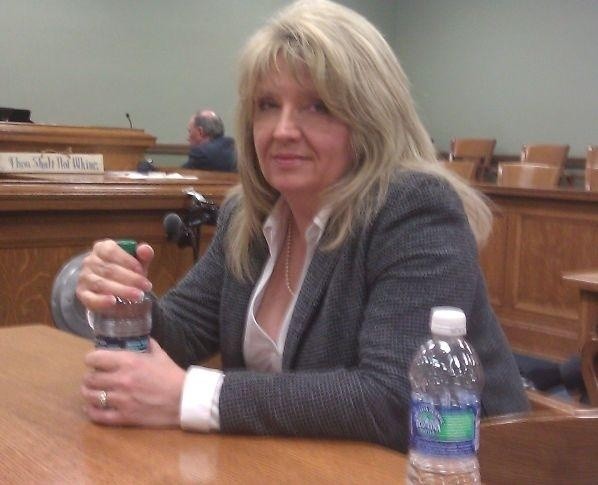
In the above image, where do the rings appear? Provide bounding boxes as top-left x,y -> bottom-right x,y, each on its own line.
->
98,389 -> 107,408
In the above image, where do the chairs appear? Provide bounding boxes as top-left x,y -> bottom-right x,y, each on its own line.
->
452,136 -> 497,180
442,155 -> 485,180
584,145 -> 598,190
0,121 -> 156,171
495,162 -> 563,189
520,144 -> 570,166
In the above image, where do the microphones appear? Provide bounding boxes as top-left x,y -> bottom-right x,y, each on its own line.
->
126,113 -> 132,128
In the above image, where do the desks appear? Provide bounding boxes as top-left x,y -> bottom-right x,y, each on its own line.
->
562,269 -> 598,409
0,325 -> 409,484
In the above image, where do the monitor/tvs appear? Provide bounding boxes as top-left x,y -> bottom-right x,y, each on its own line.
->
0,107 -> 31,123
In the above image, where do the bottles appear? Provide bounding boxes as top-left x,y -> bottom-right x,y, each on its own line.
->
92,239 -> 158,356
405,302 -> 487,485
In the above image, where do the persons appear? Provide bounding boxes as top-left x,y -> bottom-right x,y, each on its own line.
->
72,0 -> 534,457
179,108 -> 238,174
